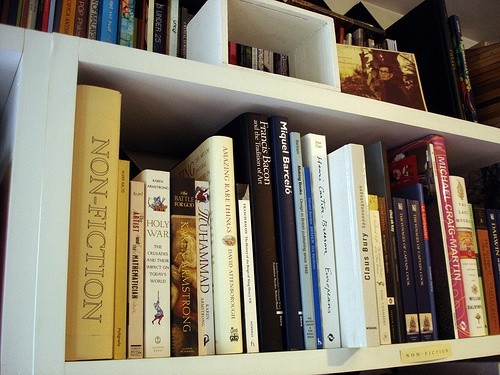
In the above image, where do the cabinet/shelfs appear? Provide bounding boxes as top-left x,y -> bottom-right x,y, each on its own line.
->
0,0 -> 500,375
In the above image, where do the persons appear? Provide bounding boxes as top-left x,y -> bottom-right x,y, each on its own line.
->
378,65 -> 413,108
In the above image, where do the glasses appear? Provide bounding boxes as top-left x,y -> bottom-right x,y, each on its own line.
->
379,70 -> 391,74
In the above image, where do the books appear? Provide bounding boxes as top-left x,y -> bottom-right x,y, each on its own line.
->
0,0 -> 500,131
64,84 -> 500,361
